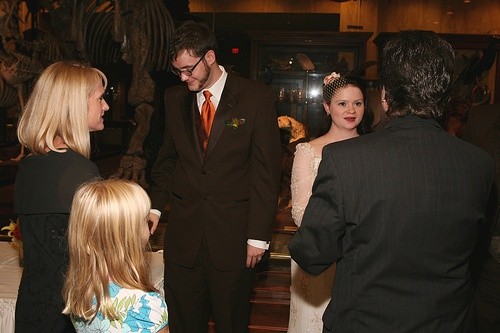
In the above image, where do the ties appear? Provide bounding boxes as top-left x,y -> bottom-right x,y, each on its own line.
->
201,90 -> 216,160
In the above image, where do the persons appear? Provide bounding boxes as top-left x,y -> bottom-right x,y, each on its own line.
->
289,72 -> 365,333
143,20 -> 280,333
63,178 -> 169,333
287,34 -> 500,333
12,60 -> 109,332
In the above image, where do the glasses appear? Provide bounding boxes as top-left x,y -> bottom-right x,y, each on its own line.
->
170,56 -> 204,75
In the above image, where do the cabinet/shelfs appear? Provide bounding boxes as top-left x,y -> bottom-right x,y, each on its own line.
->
247,30 -> 374,255
